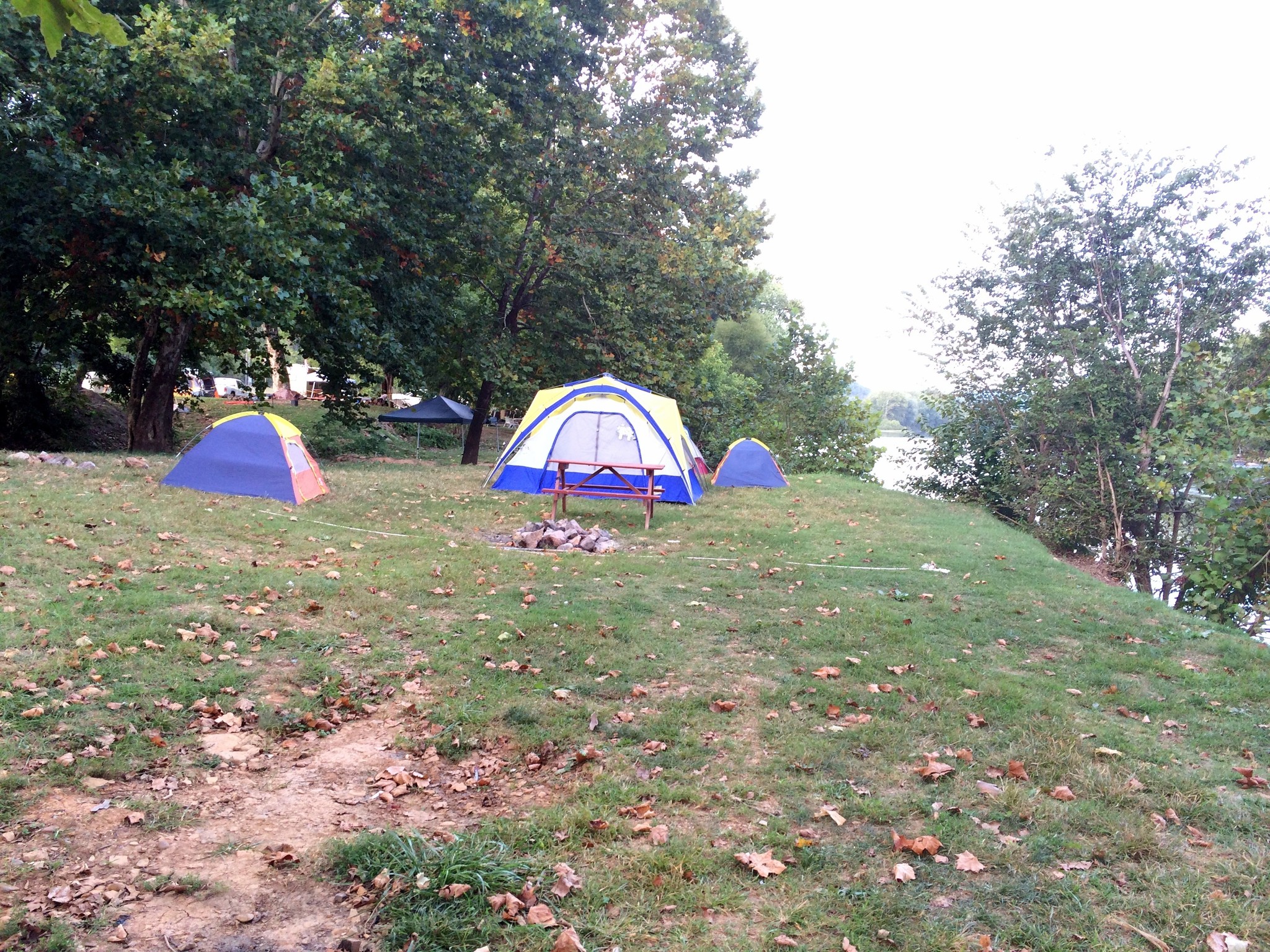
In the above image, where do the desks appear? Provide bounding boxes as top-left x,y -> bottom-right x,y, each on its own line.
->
514,418 -> 523,421
312,389 -> 335,399
546,459 -> 666,530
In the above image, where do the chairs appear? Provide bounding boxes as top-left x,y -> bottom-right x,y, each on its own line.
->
503,417 -> 519,430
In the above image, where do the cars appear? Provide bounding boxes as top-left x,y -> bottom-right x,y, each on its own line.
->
214,377 -> 253,400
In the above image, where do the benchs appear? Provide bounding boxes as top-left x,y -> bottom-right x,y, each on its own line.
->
541,488 -> 661,500
307,397 -> 336,401
554,483 -> 665,494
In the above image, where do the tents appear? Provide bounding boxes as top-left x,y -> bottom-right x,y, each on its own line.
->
710,436 -> 791,489
680,426 -> 713,476
482,373 -> 705,506
159,410 -> 332,505
375,394 -> 485,459
